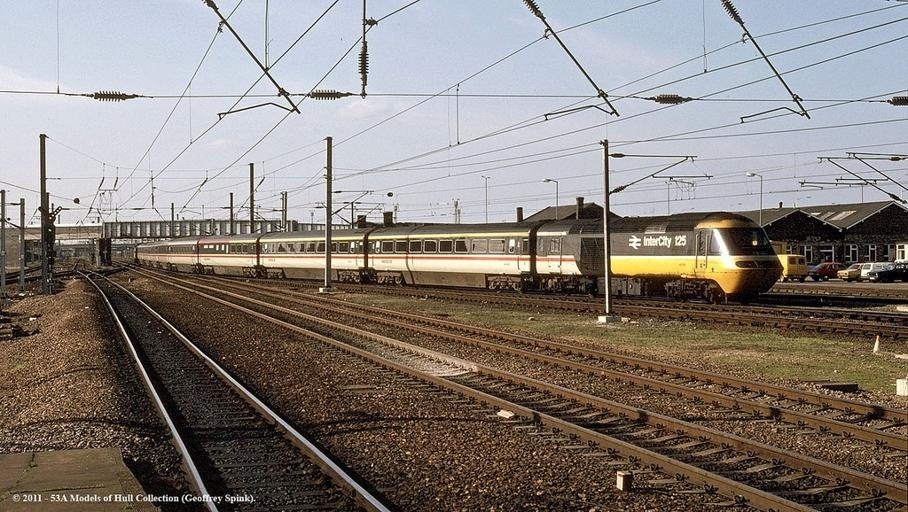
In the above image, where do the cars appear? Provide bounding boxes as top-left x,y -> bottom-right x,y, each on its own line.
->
774,252 -> 908,286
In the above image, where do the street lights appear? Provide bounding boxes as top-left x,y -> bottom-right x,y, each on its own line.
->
746,171 -> 765,230
541,178 -> 561,221
481,176 -> 493,224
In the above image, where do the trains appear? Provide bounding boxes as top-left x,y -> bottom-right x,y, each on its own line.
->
130,209 -> 787,309
